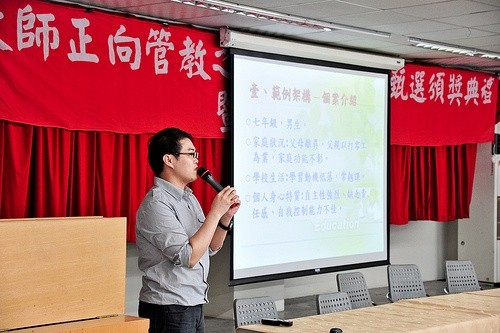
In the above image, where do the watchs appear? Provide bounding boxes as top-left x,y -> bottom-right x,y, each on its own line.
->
218,221 -> 234,230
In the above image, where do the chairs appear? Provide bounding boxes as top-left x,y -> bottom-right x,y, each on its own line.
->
233,295 -> 279,329
387,263 -> 429,304
444,259 -> 484,295
316,291 -> 352,314
336,271 -> 377,310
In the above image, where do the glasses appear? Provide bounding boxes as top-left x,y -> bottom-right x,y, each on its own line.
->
173,152 -> 199,159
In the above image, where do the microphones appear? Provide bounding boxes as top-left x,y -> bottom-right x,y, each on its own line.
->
197,167 -> 224,193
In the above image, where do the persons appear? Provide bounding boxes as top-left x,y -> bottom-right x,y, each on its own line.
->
137,127 -> 241,333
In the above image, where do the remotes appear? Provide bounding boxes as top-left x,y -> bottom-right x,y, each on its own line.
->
261,318 -> 294,326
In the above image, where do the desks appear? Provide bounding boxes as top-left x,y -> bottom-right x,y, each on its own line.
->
235,287 -> 500,333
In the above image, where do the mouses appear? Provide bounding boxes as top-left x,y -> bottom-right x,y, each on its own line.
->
330,328 -> 343,333
